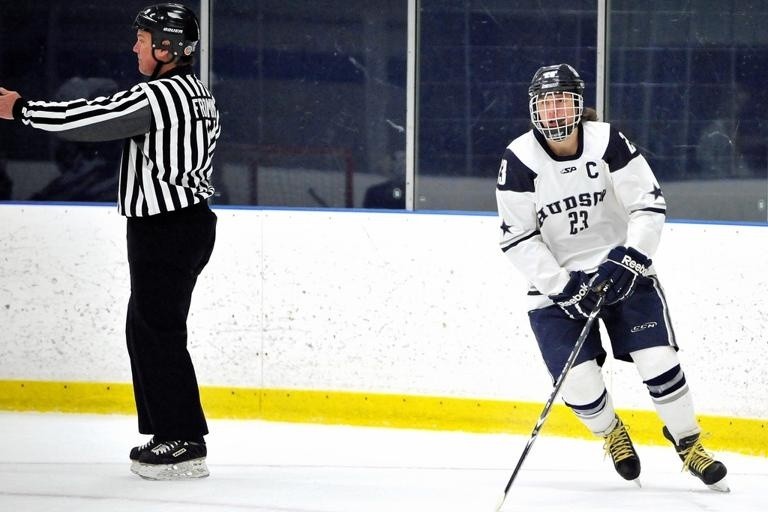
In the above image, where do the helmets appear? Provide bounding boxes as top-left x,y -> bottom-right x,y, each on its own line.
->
131,2 -> 201,57
528,64 -> 586,140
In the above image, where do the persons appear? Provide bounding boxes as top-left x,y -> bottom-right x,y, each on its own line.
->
488,60 -> 734,486
696,79 -> 753,179
31,134 -> 117,201
0,2 -> 222,465
356,145 -> 406,209
52,57 -> 119,104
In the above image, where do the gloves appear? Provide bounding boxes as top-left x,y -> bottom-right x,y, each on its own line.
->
542,270 -> 600,323
588,245 -> 654,308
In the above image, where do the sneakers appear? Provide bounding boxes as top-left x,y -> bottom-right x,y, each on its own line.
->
660,425 -> 729,486
600,415 -> 641,482
128,434 -> 208,465
